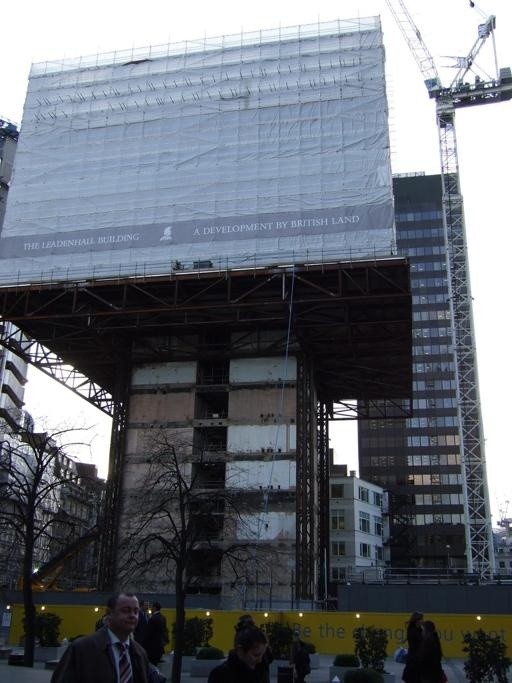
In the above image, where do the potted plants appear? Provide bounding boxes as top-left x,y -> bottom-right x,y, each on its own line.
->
263,621 -> 322,676
27,612 -> 67,661
173,616 -> 229,679
325,625 -> 395,682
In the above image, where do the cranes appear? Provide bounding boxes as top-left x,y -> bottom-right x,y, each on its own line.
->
384,1 -> 512,581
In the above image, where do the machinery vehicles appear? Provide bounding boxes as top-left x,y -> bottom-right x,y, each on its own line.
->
15,524 -> 99,593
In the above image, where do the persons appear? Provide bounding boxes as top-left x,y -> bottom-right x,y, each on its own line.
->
208,623 -> 270,683
52,591 -> 170,683
402,611 -> 430,682
292,631 -> 311,683
423,621 -> 446,681
